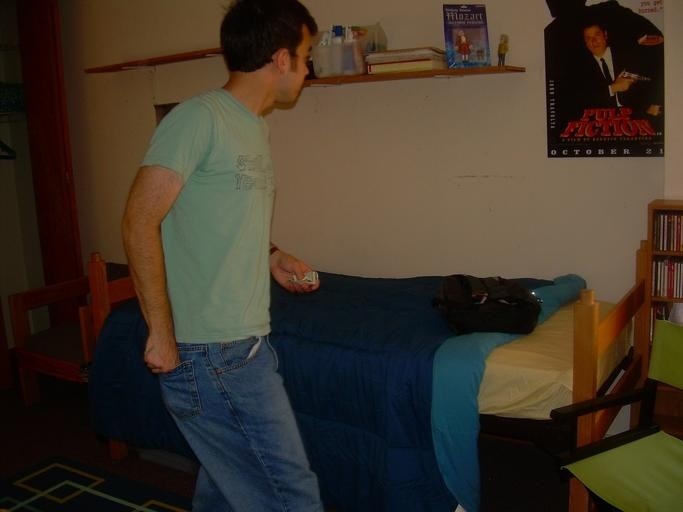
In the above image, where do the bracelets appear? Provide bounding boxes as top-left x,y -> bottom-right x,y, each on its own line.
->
268,247 -> 279,254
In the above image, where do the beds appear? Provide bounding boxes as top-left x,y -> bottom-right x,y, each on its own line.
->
88,238 -> 655,512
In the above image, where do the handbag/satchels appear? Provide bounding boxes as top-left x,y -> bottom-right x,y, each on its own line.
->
433,273 -> 540,337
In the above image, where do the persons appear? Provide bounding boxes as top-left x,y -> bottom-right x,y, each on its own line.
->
122,0 -> 326,512
454,29 -> 471,61
574,22 -> 662,118
498,35 -> 509,66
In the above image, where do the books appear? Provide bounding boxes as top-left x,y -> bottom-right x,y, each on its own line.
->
366,46 -> 447,74
652,258 -> 683,300
653,213 -> 683,253
649,304 -> 667,342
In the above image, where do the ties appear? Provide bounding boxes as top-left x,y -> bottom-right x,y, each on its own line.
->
600,57 -> 618,108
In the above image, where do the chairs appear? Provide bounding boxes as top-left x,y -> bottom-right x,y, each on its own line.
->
7,258 -> 136,410
547,317 -> 683,512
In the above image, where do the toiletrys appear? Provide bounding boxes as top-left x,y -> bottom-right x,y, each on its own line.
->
331,23 -> 343,77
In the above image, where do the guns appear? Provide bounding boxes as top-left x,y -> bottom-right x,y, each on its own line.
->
623,72 -> 651,82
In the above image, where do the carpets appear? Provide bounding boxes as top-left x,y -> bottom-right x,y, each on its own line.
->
0,450 -> 195,512
